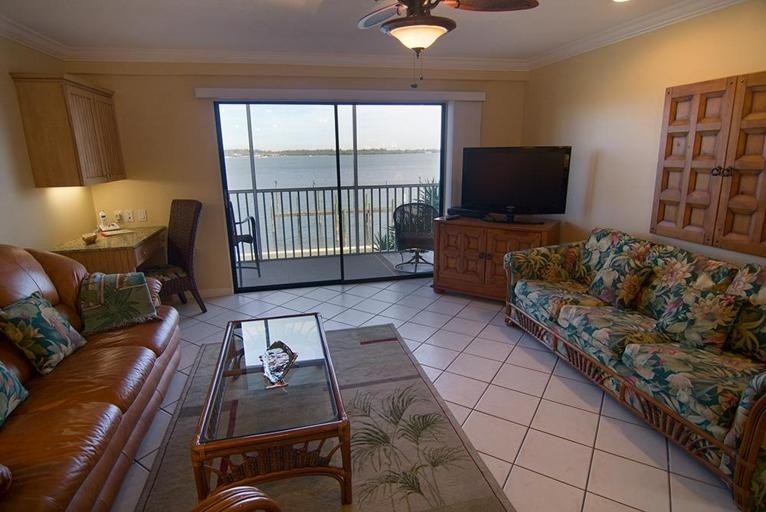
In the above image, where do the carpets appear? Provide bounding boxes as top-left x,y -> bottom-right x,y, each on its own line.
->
130,322 -> 519,511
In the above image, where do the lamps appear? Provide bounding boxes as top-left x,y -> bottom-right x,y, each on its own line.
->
376,1 -> 456,58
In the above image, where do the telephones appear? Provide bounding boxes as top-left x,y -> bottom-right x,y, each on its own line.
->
98,210 -> 121,232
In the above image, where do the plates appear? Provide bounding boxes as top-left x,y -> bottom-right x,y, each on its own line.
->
98,224 -> 119,231
259,340 -> 299,384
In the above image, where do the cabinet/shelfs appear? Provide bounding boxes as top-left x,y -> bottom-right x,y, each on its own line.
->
13,72 -> 129,191
427,210 -> 561,309
644,70 -> 766,256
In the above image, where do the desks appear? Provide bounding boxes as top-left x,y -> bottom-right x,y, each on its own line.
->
50,226 -> 168,281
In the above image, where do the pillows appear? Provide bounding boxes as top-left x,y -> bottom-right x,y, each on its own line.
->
655,281 -> 745,358
0,360 -> 33,427
0,290 -> 88,374
586,247 -> 653,310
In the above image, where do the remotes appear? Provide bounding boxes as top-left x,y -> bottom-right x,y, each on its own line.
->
446,215 -> 459,220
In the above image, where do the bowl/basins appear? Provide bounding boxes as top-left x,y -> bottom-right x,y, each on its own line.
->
81,233 -> 96,245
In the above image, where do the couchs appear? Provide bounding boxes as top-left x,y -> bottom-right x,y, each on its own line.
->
498,225 -> 764,511
0,242 -> 185,511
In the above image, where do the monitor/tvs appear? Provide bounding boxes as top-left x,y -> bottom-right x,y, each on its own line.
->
461,146 -> 572,225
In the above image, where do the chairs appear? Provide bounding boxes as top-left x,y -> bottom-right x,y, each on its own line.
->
192,484 -> 281,511
138,194 -> 207,322
393,203 -> 440,276
223,200 -> 264,282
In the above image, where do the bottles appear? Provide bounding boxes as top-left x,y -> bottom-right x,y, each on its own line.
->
99,208 -> 107,229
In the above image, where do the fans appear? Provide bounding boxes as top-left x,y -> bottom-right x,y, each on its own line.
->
357,1 -> 540,35
